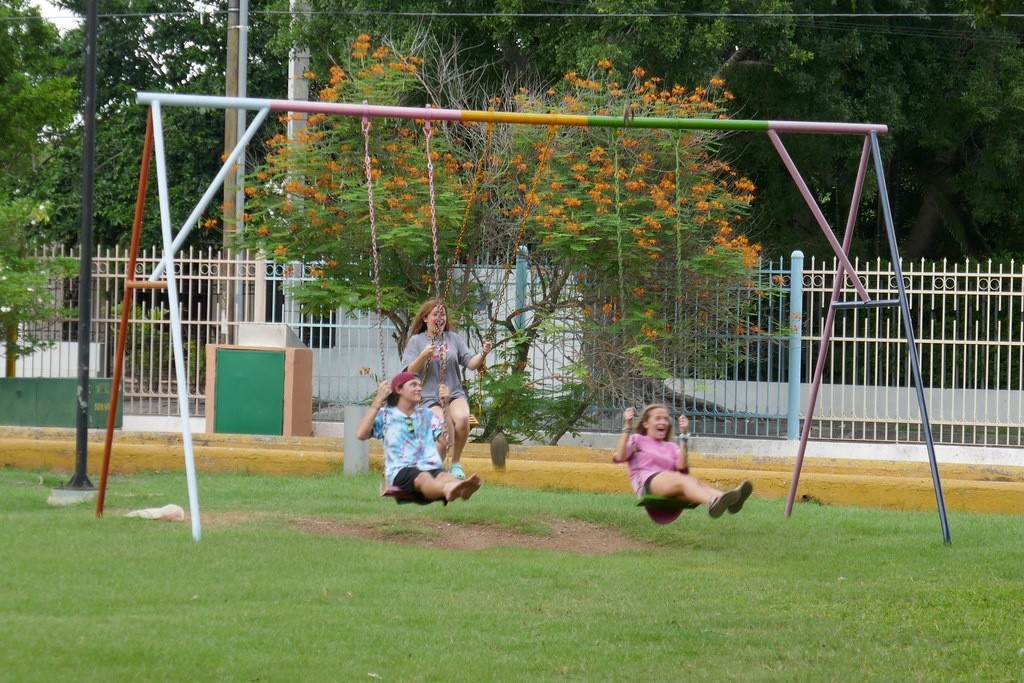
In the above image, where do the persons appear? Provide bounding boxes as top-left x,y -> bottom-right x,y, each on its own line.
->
610,402 -> 754,524
355,372 -> 484,507
400,296 -> 492,480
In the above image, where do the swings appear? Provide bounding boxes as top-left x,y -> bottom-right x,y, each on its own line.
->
358,97 -> 453,500
607,123 -> 704,511
415,120 -> 560,431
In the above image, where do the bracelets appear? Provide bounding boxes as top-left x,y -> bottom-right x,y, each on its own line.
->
370,403 -> 380,410
680,432 -> 690,443
480,351 -> 485,358
621,427 -> 632,433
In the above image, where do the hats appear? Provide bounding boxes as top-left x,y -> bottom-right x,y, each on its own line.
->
389,370 -> 420,407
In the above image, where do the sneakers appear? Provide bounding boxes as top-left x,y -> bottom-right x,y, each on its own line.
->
709,482 -> 752,519
451,464 -> 466,480
